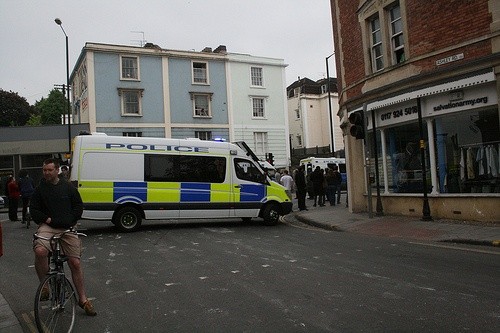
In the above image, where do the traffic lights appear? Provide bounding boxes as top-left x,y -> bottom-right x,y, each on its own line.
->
269,152 -> 276,166
348,109 -> 365,139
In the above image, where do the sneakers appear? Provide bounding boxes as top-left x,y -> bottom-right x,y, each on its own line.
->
78,298 -> 97,317
40,288 -> 50,302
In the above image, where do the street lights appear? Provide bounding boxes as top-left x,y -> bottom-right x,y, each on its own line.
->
54,18 -> 71,164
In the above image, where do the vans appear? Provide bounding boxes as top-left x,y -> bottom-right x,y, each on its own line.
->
68,130 -> 294,234
299,156 -> 347,192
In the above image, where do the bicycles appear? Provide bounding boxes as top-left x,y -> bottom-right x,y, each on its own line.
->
33,226 -> 88,333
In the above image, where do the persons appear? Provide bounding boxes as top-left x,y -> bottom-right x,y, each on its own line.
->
310,166 -> 326,207
325,169 -> 338,206
275,166 -> 342,204
58,166 -> 70,179
280,170 -> 294,212
295,166 -> 309,210
7,177 -> 21,221
19,172 -> 35,224
28,158 -> 98,316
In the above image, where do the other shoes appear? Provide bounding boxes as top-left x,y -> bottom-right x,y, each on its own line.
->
313,205 -> 317,207
294,198 -> 297,199
320,204 -> 326,207
337,202 -> 341,204
17,218 -> 21,221
307,198 -> 310,199
